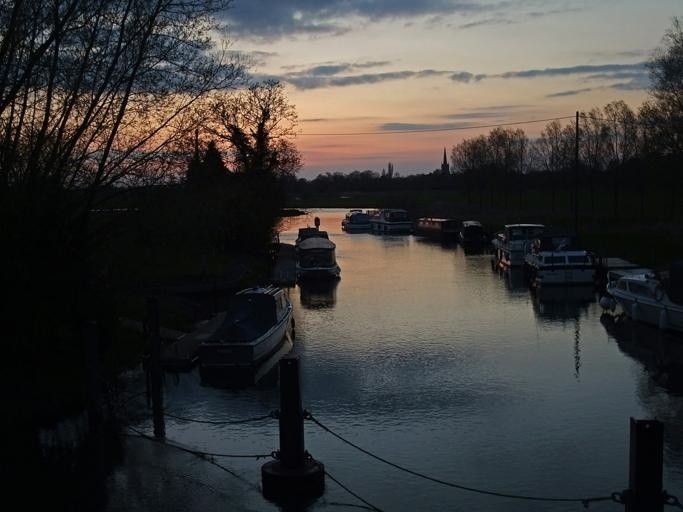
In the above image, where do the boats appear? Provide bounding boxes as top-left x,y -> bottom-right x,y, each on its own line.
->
293,216 -> 340,285
409,216 -> 461,241
342,209 -> 371,233
368,208 -> 416,237
491,223 -> 556,276
524,235 -> 598,294
459,219 -> 493,250
598,266 -> 681,334
195,284 -> 295,384
